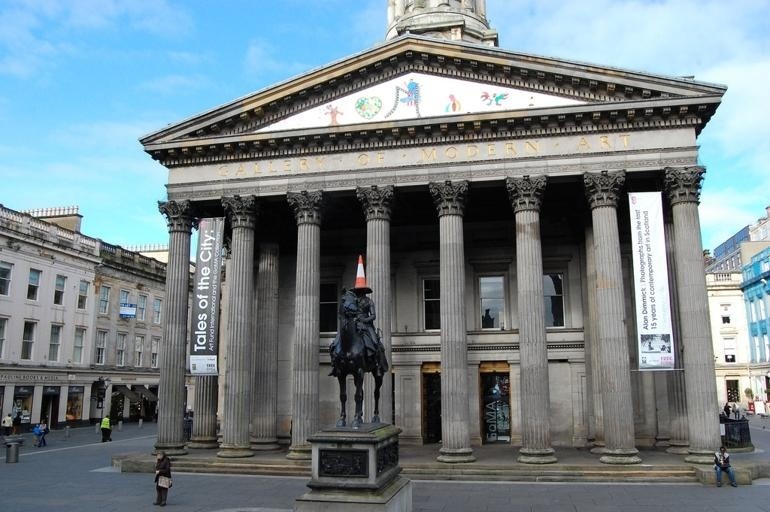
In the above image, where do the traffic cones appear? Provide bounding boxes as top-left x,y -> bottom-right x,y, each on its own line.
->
349,255 -> 373,293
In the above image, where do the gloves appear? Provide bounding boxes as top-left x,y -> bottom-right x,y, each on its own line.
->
156,471 -> 159,474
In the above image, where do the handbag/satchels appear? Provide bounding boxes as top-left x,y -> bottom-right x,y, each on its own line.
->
158,475 -> 171,488
44,428 -> 50,434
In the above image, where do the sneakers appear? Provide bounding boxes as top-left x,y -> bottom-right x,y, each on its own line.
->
717,482 -> 721,486
154,501 -> 165,506
732,483 -> 737,486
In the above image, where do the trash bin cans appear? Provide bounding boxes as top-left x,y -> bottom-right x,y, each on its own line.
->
6,443 -> 20,463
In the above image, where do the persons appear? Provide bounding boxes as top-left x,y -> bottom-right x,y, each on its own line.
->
31,424 -> 42,448
184,410 -> 193,441
100,415 -> 112,443
37,420 -> 47,448
732,401 -> 740,419
724,402 -> 731,418
13,412 -> 23,436
325,290 -> 388,381
713,447 -> 738,489
153,450 -> 171,508
2,413 -> 13,436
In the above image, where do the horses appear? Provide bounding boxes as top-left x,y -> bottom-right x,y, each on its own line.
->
332,286 -> 386,429
